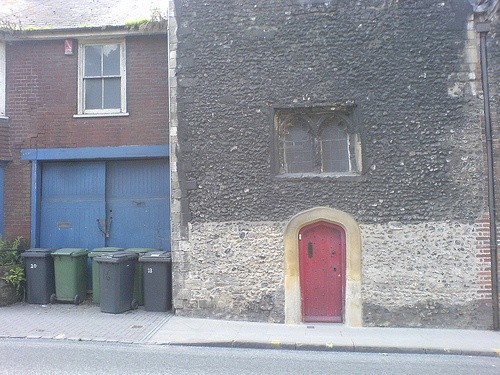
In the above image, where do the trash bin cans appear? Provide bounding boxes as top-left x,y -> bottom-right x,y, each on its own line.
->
87,247 -> 124,304
126,247 -> 163,306
138,250 -> 172,312
92,250 -> 139,315
21,247 -> 53,304
50,247 -> 90,305
